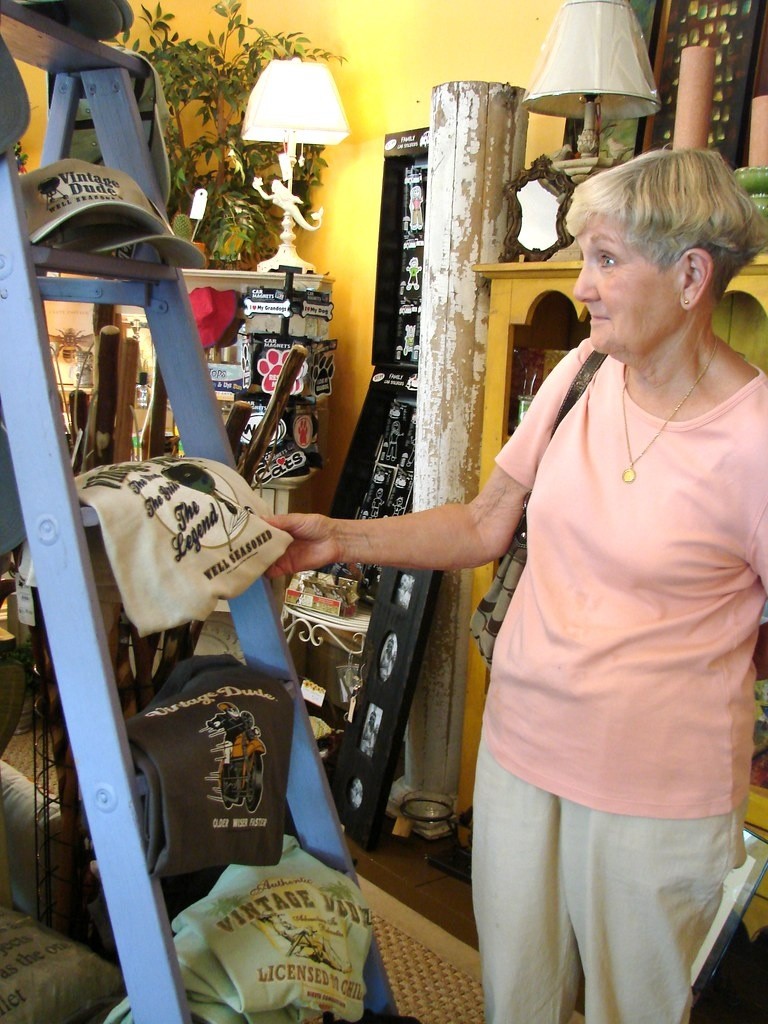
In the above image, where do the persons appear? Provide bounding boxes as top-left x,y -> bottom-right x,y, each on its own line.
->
258,147 -> 767,1024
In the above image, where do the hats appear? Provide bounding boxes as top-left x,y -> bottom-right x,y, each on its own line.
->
188,286 -> 239,350
0,0 -> 211,272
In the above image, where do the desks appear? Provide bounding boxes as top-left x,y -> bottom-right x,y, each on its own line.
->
280,600 -> 371,656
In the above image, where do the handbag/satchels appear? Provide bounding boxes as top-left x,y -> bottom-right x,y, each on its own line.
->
472,349 -> 607,666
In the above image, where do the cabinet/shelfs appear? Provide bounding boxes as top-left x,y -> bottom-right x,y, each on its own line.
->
116,268 -> 336,678
456,253 -> 768,940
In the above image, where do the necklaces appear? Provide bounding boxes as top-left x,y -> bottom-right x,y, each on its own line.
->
619,332 -> 726,483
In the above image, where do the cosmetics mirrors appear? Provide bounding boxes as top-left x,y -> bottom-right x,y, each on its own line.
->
501,152 -> 578,261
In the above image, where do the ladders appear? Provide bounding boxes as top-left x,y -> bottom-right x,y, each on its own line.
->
0,0 -> 399,1024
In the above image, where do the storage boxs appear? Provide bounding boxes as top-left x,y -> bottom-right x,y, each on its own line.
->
284,570 -> 359,618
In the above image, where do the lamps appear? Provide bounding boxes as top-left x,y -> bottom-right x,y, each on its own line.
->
239,57 -> 352,274
520,0 -> 662,159
